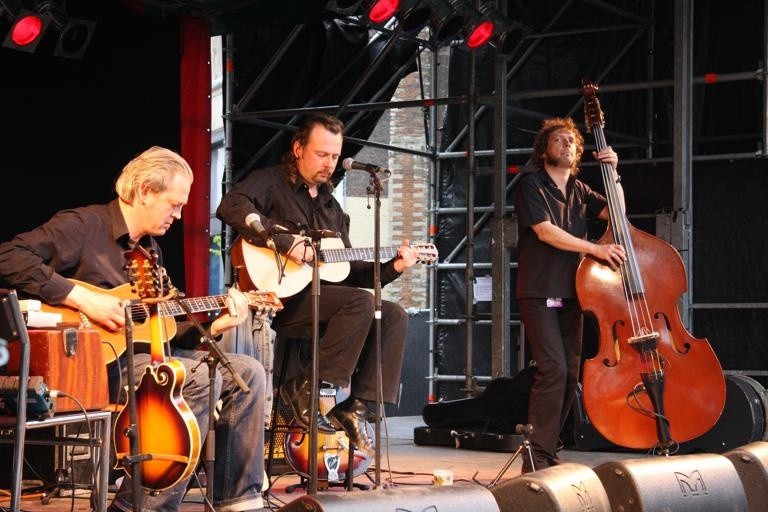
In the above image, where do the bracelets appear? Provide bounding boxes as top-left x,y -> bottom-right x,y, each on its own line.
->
616,175 -> 621,183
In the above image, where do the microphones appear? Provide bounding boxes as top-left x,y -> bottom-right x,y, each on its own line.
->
244,211 -> 278,253
155,264 -> 172,292
340,155 -> 392,180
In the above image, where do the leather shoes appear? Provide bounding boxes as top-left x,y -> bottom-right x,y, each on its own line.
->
279,374 -> 336,435
325,396 -> 382,459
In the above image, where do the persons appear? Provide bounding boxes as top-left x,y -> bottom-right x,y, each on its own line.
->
511,114 -> 628,474
2,143 -> 267,511
213,109 -> 418,459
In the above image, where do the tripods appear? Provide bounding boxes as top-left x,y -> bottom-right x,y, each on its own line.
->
487,443 -> 540,486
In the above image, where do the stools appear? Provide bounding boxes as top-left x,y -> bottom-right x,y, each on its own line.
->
263,326 -> 356,497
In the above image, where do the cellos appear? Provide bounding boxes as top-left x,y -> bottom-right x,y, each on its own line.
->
575,83 -> 727,449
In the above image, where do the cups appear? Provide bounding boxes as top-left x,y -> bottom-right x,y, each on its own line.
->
432,469 -> 455,487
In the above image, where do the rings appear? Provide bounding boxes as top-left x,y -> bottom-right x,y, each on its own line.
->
612,253 -> 615,258
230,313 -> 237,318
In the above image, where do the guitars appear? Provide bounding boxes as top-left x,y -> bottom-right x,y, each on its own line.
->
284,372 -> 375,481
41,278 -> 284,366
229,221 -> 438,299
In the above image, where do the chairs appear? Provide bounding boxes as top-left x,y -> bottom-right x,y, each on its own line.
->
0,288 -> 113,511
